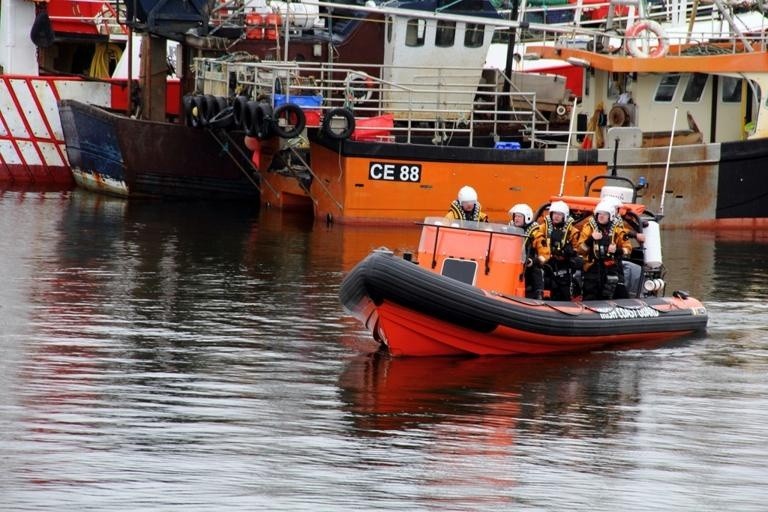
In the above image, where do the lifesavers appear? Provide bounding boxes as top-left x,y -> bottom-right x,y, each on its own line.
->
626,20 -> 665,59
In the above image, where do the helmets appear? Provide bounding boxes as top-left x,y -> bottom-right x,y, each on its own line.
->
458,186 -> 478,206
509,204 -> 533,224
550,201 -> 569,222
594,197 -> 622,222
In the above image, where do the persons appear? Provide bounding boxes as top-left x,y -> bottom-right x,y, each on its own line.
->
503,202 -> 542,299
575,199 -> 632,297
443,185 -> 491,229
505,351 -> 655,442
535,200 -> 582,301
610,196 -> 646,295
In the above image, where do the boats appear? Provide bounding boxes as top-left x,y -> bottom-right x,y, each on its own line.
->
330,340 -> 666,445
336,193 -> 710,351
